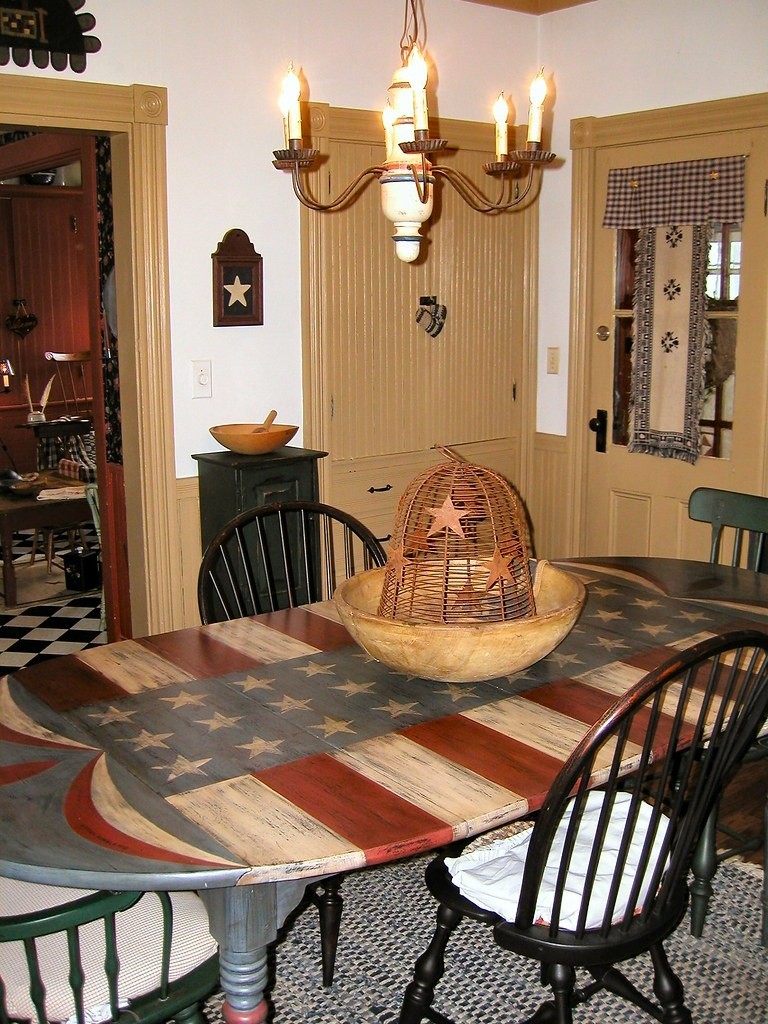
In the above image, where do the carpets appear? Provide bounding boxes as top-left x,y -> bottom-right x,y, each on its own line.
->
164,852 -> 767,1023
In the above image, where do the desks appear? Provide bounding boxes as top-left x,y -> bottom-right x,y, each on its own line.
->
0,556 -> 768,1024
14,415 -> 91,469
0,468 -> 103,609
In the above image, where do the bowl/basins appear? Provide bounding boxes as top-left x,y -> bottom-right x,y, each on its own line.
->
333,556 -> 586,683
209,426 -> 300,455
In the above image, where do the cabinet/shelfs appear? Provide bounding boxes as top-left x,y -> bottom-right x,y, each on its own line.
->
191,447 -> 330,624
292,95 -> 540,461
1,400 -> 95,474
305,438 -> 529,608
0,184 -> 103,402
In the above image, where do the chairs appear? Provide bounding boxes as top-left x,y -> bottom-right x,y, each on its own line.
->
29,458 -> 90,575
85,482 -> 107,632
1,876 -> 221,1024
44,351 -> 93,415
615,486 -> 768,950
398,630 -> 768,1024
196,501 -> 390,987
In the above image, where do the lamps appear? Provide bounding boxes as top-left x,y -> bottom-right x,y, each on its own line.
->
272,0 -> 556,262
